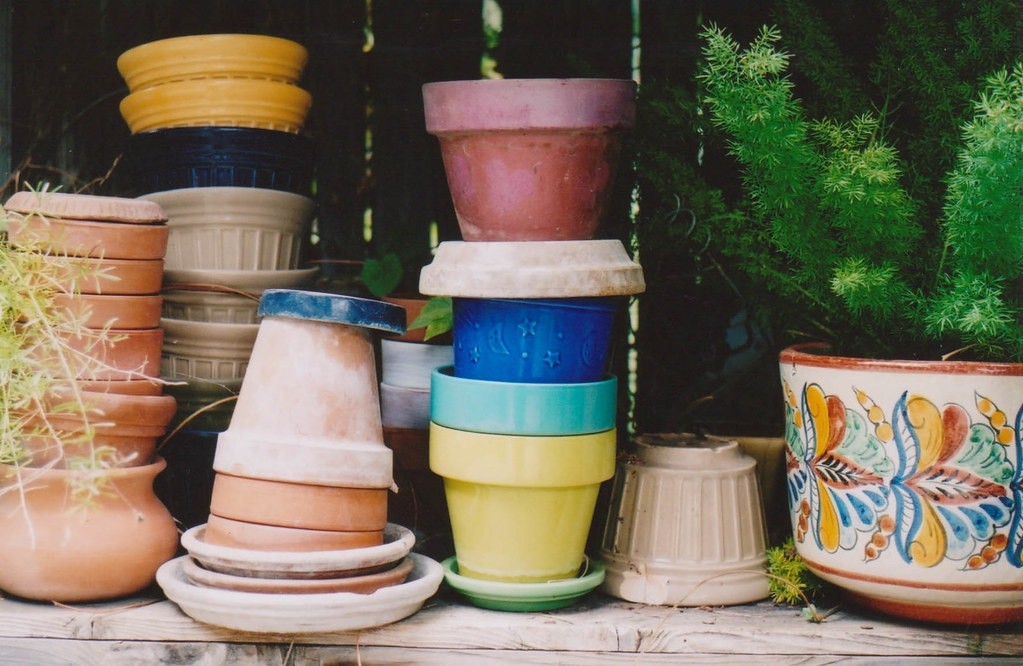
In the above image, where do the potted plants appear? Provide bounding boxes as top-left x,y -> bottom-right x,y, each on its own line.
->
637,0 -> 1023,631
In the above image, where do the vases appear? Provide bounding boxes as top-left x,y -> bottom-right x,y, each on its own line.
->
0,33 -> 782,640
